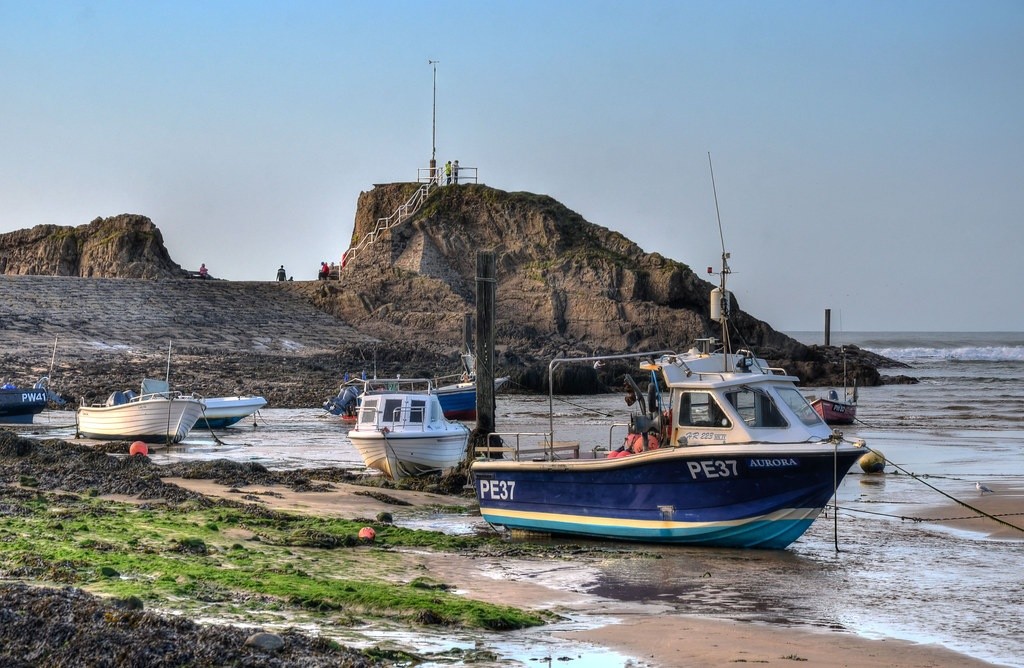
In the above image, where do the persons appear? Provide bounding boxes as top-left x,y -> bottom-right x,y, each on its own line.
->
319,262 -> 334,281
277,265 -> 293,281
200,264 -> 208,280
446,160 -> 458,185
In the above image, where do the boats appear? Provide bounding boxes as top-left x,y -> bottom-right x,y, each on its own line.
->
347,391 -> 472,481
469,149 -> 871,550
322,386 -> 480,421
190,394 -> 269,429
0,376 -> 50,425
798,355 -> 861,424
421,371 -> 513,392
76,378 -> 208,446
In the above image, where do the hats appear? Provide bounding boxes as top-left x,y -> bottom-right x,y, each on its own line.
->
321,262 -> 324,266
